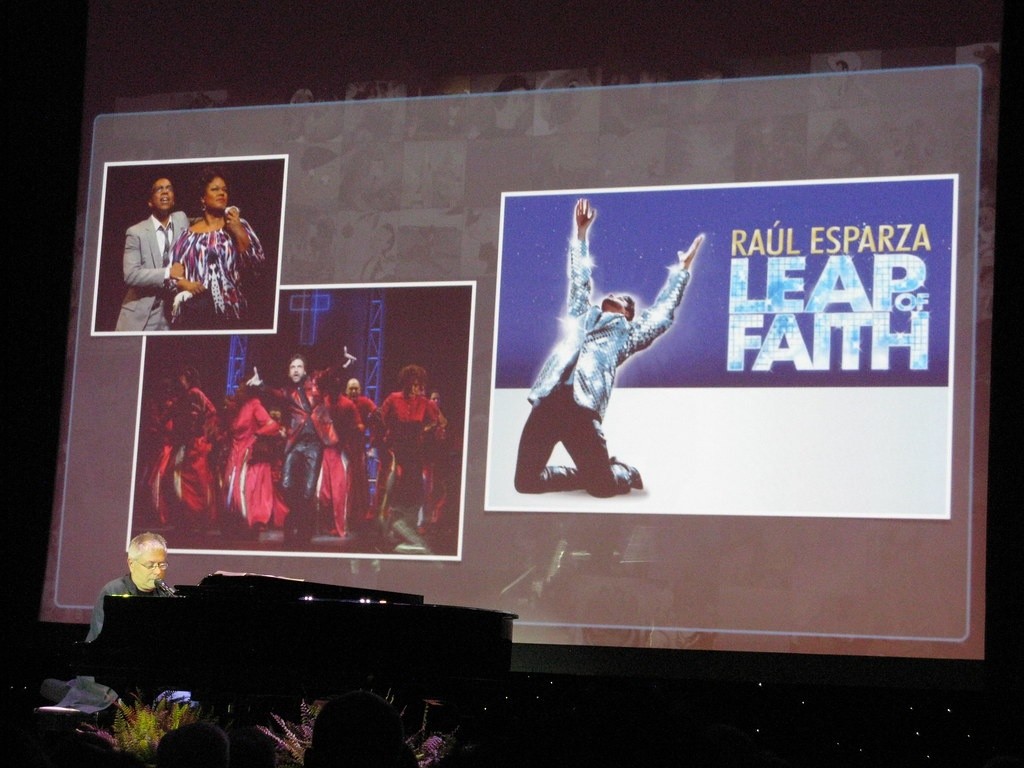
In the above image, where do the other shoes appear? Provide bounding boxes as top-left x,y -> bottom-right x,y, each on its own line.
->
608,456 -> 642,489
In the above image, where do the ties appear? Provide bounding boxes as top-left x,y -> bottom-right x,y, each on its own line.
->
152,223 -> 173,312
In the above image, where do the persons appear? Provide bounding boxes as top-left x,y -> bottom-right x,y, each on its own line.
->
85,532 -> 179,643
0,721 -> 276,768
514,198 -> 704,498
303,691 -> 418,768
115,172 -> 266,330
133,344 -> 462,553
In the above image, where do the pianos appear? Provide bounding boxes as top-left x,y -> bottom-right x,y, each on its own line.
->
71,569 -> 520,695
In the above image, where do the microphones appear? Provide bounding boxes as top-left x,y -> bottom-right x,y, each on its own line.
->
154,578 -> 173,598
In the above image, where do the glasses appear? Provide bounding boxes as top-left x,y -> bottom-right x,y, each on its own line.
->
131,560 -> 169,571
